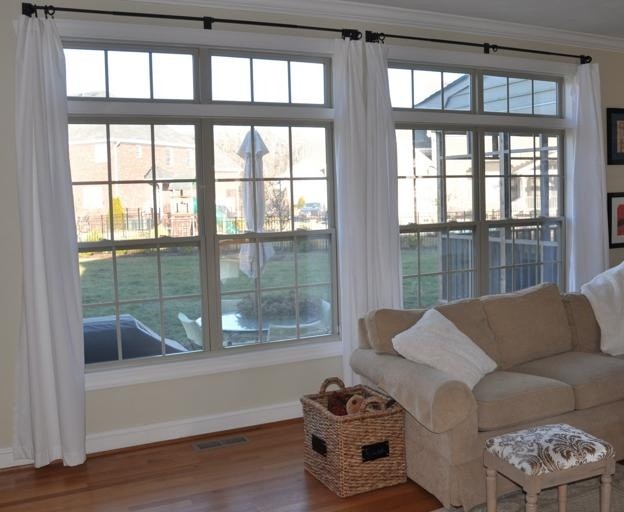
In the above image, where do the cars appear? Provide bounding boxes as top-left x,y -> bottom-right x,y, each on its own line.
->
298,205 -> 323,217
83,313 -> 191,365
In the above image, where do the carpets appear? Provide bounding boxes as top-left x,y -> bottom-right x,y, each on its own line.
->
435,461 -> 623,511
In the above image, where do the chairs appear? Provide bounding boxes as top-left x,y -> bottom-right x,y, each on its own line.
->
173,294 -> 333,349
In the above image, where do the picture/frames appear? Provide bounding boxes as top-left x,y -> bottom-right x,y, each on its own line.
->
607,192 -> 624,249
607,107 -> 624,166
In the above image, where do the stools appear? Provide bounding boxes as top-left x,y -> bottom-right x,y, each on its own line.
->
482,423 -> 616,511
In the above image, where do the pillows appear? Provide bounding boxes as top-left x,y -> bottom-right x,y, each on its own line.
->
389,306 -> 498,393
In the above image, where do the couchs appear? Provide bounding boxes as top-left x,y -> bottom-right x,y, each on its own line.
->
349,281 -> 623,511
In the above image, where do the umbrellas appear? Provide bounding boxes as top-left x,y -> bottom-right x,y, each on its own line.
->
232,128 -> 277,322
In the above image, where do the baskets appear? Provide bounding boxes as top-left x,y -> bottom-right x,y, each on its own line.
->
301,376 -> 408,498
